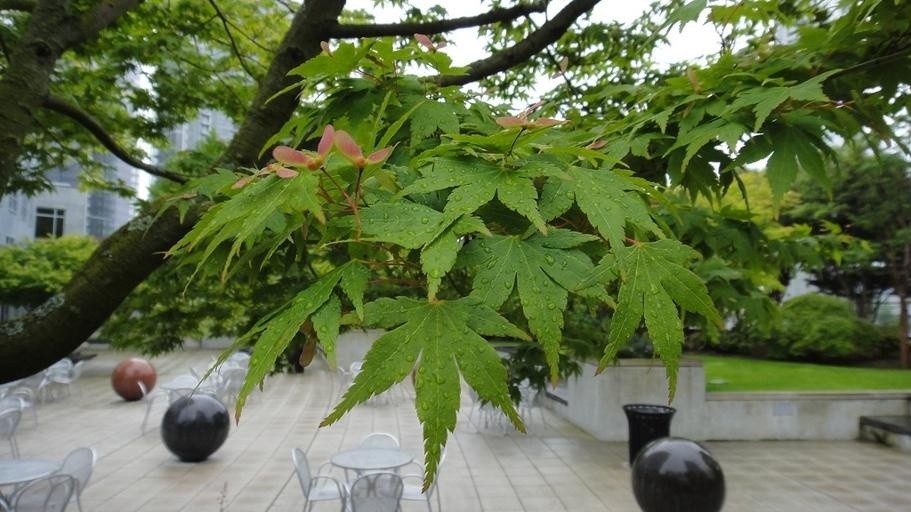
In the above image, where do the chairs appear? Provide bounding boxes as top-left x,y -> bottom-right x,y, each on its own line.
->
336,361 -> 416,406
467,383 -> 549,436
291,432 -> 447,512
1,357 -> 98,512
137,352 -> 270,435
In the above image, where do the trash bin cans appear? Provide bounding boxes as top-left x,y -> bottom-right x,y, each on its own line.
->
623,404 -> 677,468
